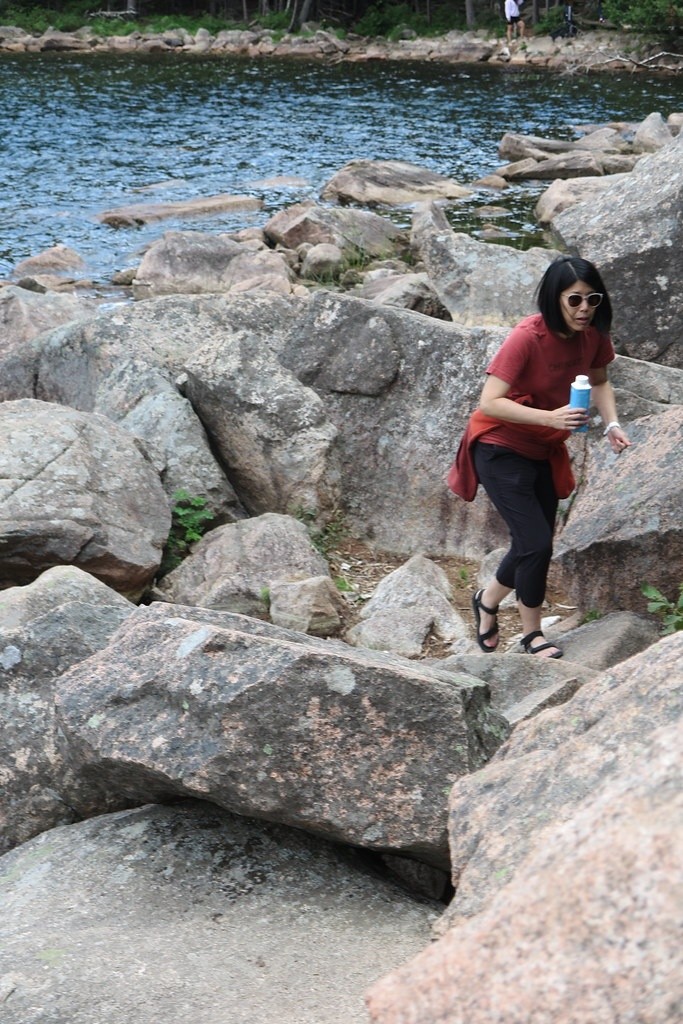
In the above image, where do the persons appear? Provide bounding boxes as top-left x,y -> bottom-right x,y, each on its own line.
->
504,0 -> 525,44
468,259 -> 630,659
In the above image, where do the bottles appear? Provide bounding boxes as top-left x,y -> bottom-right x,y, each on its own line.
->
569,375 -> 591,434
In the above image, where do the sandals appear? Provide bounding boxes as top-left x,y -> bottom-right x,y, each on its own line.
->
472,588 -> 499,652
520,630 -> 562,659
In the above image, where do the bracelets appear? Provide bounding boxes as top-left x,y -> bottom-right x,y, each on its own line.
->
603,422 -> 620,435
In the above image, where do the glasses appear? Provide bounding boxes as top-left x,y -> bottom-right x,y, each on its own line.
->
560,293 -> 604,308
